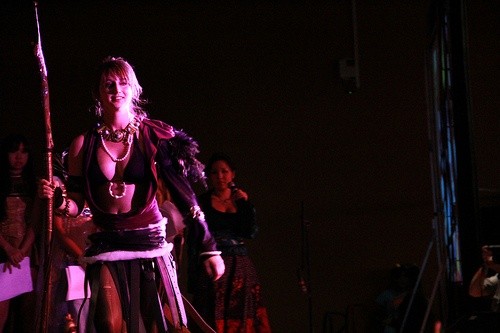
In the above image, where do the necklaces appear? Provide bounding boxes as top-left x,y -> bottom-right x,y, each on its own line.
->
100,134 -> 134,162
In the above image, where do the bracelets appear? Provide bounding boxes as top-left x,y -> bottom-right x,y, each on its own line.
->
199,250 -> 222,256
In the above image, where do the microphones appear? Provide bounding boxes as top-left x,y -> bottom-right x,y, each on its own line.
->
297,269 -> 307,292
227,182 -> 243,207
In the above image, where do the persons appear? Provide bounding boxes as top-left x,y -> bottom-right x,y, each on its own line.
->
0,129 -> 272,333
36,55 -> 226,333
467,245 -> 500,333
